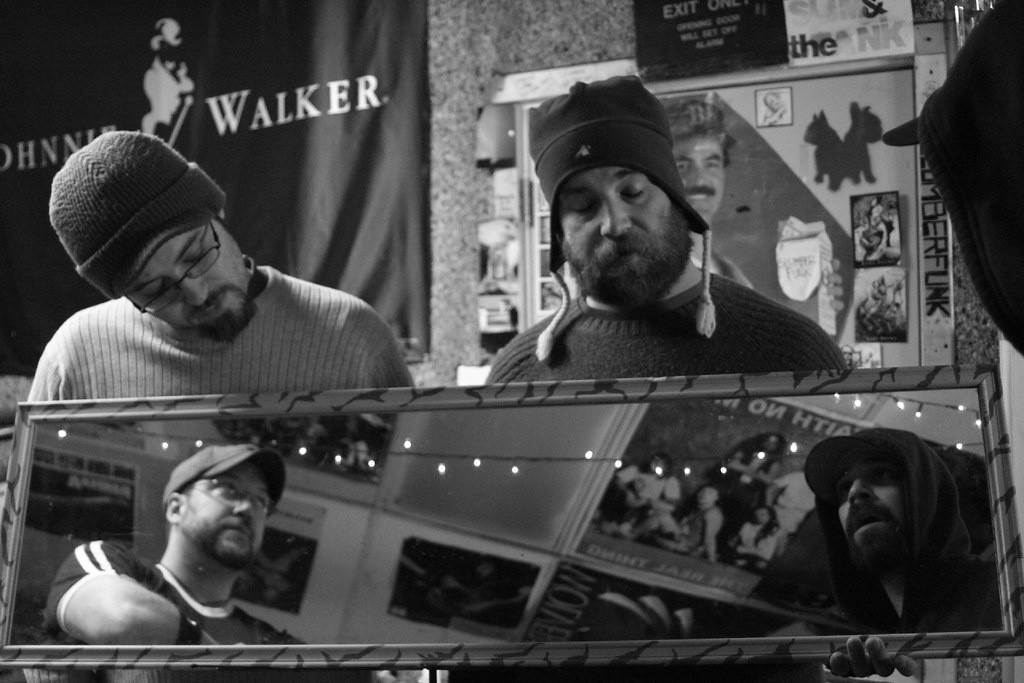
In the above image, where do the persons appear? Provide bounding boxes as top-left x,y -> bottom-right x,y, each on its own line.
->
45,444 -> 310,646
439,75 -> 917,683
662,99 -> 754,290
616,427 -> 1002,632
22,130 -> 415,683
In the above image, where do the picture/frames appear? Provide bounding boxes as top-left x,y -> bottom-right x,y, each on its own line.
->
0,364 -> 1024,670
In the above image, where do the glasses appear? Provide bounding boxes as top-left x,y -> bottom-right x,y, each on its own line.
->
211,478 -> 269,514
123,221 -> 221,314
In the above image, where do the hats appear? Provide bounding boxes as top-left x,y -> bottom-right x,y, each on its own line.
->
161,443 -> 285,506
528,74 -> 716,362
865,196 -> 885,216
804,435 -> 896,503
49,131 -> 228,300
